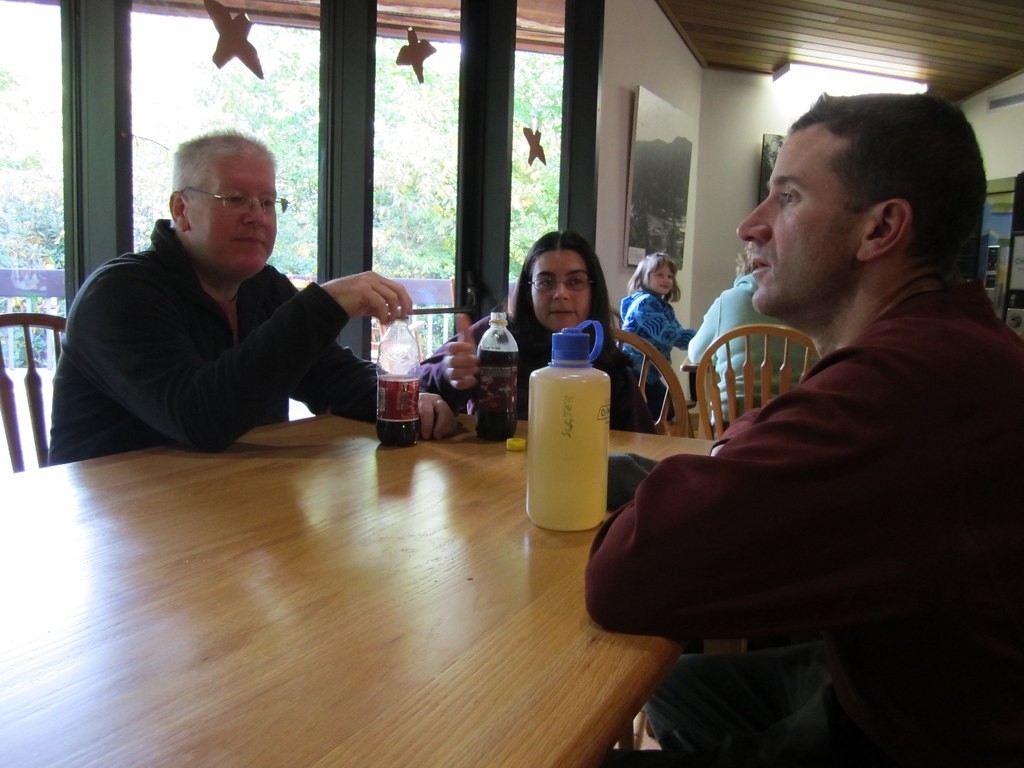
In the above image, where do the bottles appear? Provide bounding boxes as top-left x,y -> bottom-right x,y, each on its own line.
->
524,319 -> 612,532
472,311 -> 520,442
375,306 -> 422,447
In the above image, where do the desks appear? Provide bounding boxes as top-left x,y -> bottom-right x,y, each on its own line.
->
0,415 -> 724,768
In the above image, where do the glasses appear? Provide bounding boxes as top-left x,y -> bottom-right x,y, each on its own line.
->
180,186 -> 289,217
528,276 -> 594,293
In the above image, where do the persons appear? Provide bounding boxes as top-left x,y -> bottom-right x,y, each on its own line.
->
48,133 -> 460,465
616,242 -> 819,435
407,230 -> 657,435
584,94 -> 1024,768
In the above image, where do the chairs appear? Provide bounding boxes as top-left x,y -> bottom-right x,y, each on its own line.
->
609,326 -> 689,439
0,313 -> 66,474
696,324 -> 821,442
